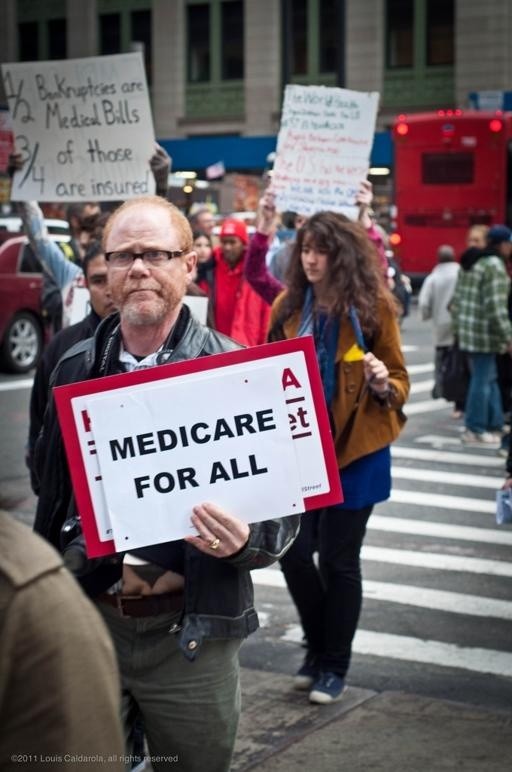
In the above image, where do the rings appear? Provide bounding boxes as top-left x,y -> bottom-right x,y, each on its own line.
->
209,538 -> 220,551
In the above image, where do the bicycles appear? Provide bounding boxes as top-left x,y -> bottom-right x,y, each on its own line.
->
394,271 -> 416,312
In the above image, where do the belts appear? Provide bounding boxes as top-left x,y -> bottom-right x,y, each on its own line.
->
96,587 -> 185,618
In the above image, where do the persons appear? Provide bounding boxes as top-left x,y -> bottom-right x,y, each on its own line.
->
2,144 -> 510,496
0,510 -> 130,769
32,195 -> 306,769
262,211 -> 411,708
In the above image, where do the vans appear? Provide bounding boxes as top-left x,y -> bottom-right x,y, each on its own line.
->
387,109 -> 510,268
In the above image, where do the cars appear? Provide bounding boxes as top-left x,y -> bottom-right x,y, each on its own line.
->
207,212 -> 262,235
3,215 -> 74,236
0,237 -> 96,363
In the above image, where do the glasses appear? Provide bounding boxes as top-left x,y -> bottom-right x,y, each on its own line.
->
104,248 -> 189,265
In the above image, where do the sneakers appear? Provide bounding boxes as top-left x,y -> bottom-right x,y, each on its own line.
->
296,649 -> 319,688
461,430 -> 499,445
309,670 -> 349,703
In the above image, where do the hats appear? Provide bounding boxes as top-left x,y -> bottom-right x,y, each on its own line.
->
218,217 -> 249,246
